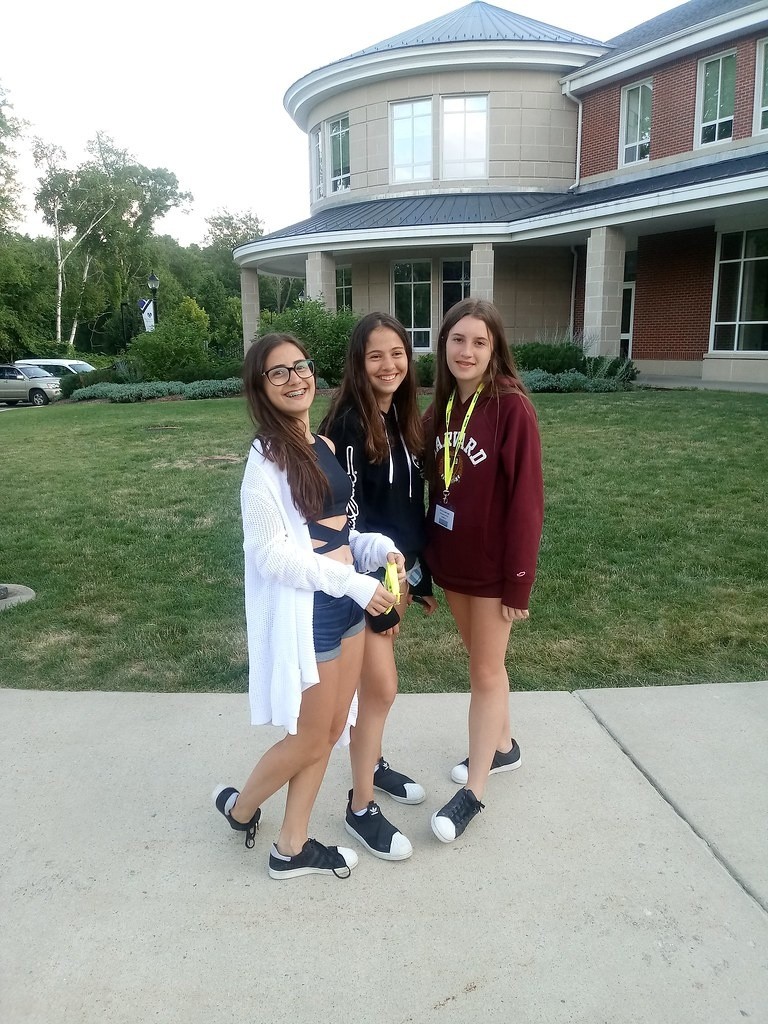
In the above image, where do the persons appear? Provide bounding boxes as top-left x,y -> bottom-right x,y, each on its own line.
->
315,312 -> 426,860
422,297 -> 544,843
211,333 -> 359,881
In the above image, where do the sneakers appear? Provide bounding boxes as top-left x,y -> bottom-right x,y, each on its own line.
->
431,786 -> 485,843
373,757 -> 426,804
268,838 -> 359,878
344,788 -> 413,860
451,738 -> 522,784
212,785 -> 262,849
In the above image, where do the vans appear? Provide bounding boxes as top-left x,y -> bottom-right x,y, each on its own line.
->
14,359 -> 96,377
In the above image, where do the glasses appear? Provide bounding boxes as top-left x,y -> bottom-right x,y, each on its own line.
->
261,358 -> 314,387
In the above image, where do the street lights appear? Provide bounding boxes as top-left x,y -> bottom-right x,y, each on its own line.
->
148,269 -> 159,330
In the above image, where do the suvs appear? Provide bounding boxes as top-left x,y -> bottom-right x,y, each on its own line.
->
0,364 -> 62,406
